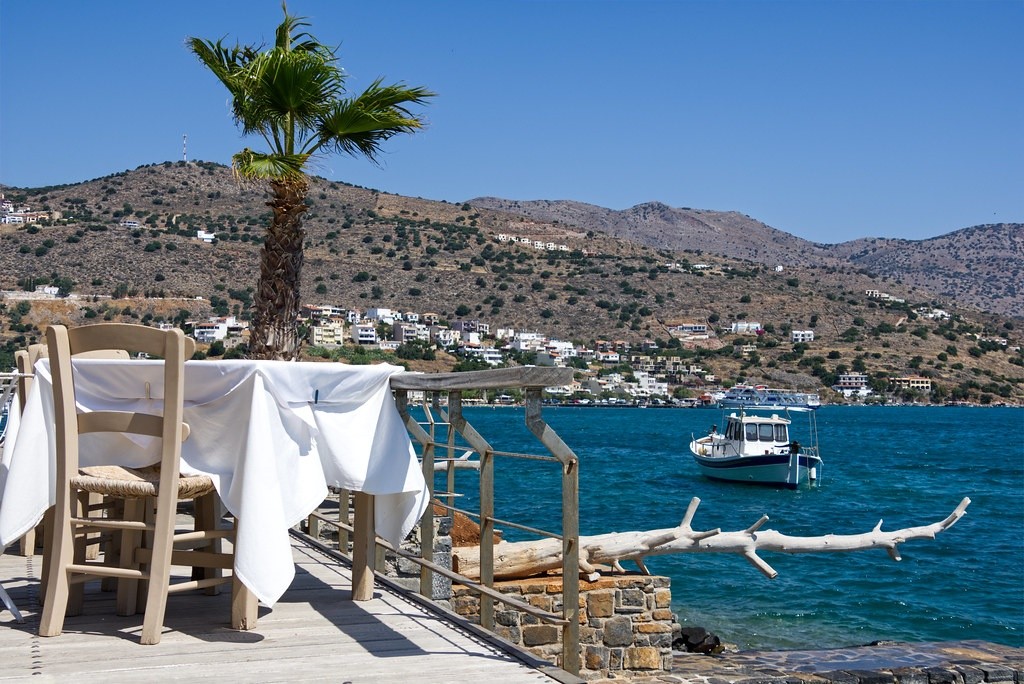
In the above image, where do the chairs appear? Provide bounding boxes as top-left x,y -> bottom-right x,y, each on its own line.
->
14,343 -> 210,602
40,333 -> 257,645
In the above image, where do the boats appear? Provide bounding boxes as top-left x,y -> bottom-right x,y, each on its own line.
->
688,402 -> 826,490
715,381 -> 823,409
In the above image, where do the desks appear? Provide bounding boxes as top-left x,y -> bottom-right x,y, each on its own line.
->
0,359 -> 430,632
